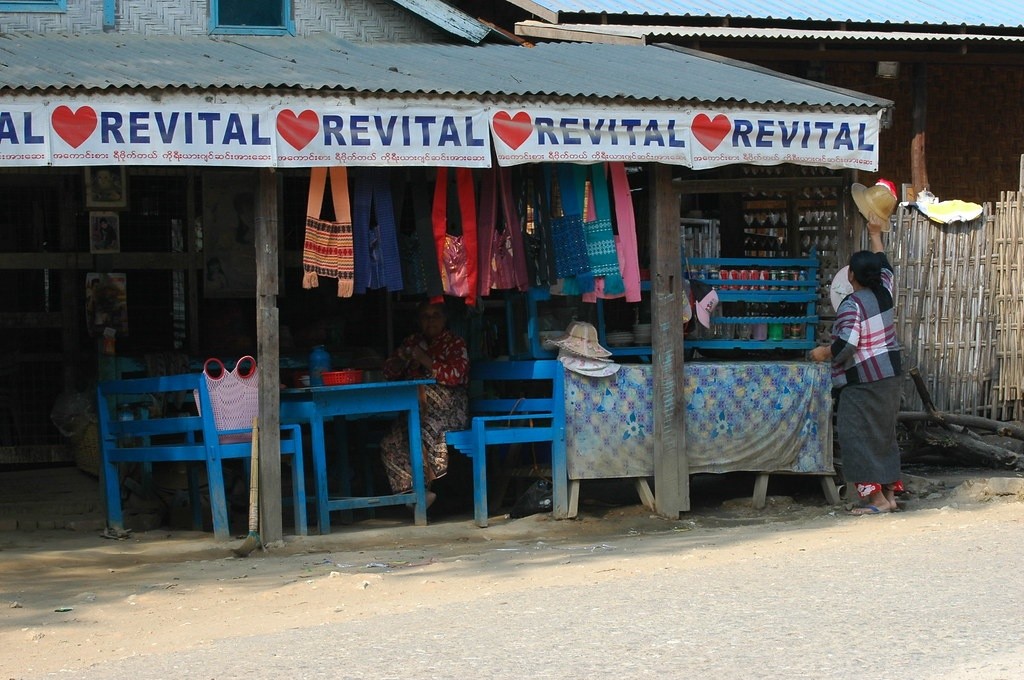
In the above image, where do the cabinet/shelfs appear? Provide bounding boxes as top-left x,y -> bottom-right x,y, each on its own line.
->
501,245 -> 821,362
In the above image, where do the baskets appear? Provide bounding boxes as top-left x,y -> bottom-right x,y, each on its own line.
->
322,370 -> 364,385
193,355 -> 260,445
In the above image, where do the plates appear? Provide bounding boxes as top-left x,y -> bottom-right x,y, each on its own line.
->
606,324 -> 652,347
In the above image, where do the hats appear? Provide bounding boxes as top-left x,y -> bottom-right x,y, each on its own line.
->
830,264 -> 854,312
689,279 -> 719,330
556,347 -> 622,378
544,320 -> 613,358
682,289 -> 692,324
851,182 -> 897,233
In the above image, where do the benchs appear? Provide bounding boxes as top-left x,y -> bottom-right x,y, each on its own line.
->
354,359 -> 570,528
95,370 -> 311,537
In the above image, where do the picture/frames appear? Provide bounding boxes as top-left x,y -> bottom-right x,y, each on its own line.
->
84,165 -> 128,207
89,211 -> 121,254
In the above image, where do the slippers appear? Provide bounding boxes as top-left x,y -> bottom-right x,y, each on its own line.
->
848,505 -> 901,515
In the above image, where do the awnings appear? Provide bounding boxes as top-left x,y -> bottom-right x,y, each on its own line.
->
1,41 -> 893,167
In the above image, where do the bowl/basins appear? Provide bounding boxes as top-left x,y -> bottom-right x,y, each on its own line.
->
298,376 -> 310,387
523,331 -> 566,352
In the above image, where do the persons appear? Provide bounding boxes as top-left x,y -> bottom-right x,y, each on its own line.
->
808,249 -> 906,514
382,298 -> 469,515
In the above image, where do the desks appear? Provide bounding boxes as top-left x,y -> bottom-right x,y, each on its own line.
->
277,379 -> 436,532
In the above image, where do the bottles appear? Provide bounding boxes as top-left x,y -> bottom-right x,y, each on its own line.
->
308,345 -> 332,387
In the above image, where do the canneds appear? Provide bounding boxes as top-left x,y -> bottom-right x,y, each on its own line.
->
689,268 -> 808,291
790,322 -> 801,339
